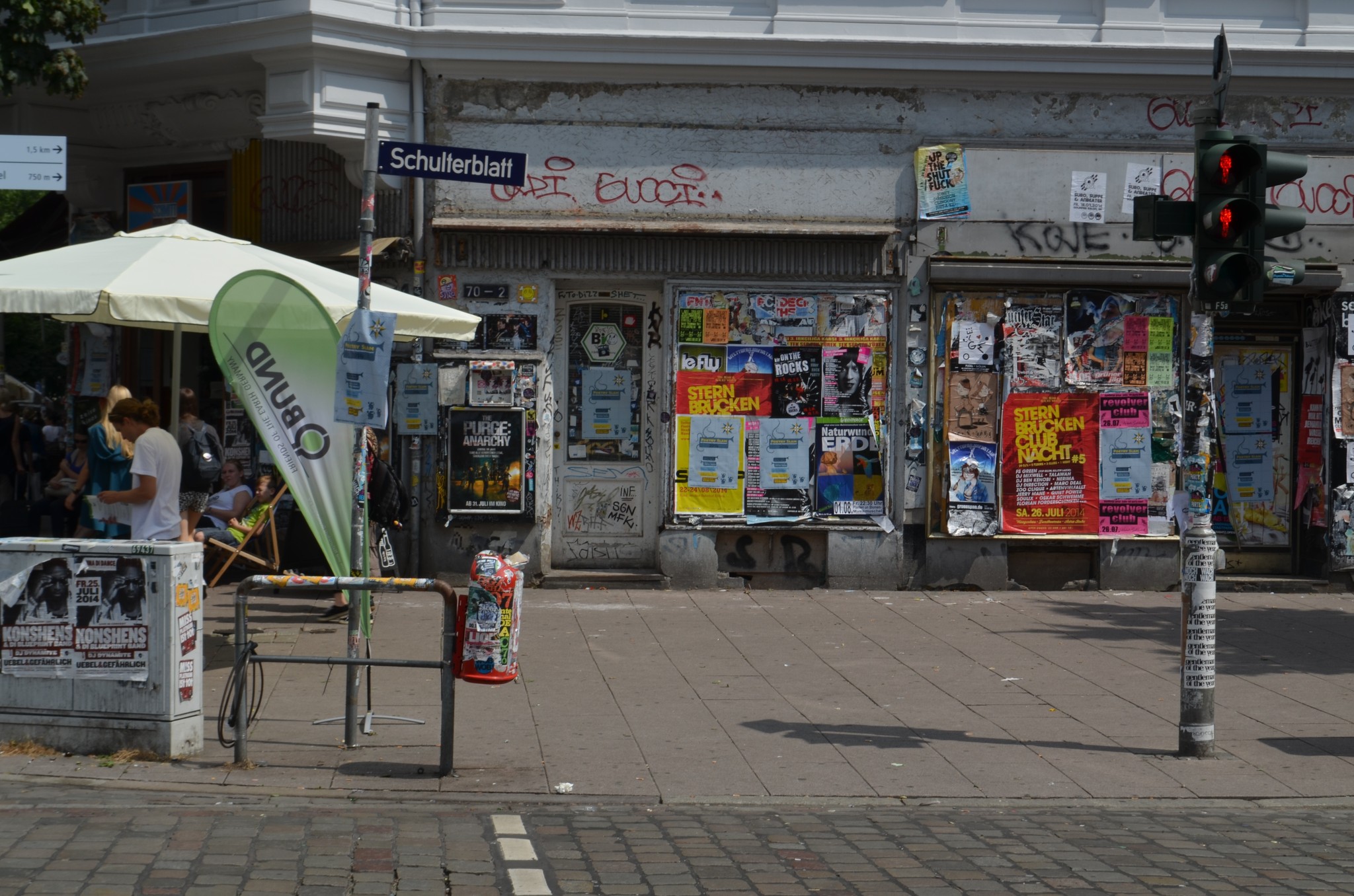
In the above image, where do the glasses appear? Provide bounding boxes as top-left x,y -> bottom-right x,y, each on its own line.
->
71,438 -> 88,444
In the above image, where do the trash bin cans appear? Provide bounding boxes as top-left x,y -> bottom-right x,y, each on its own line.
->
457,550 -> 526,682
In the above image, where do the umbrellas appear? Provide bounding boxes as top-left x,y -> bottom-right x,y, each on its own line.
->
0,218 -> 481,440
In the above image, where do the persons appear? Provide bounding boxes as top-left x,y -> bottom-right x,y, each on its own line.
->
97,397 -> 182,541
88,565 -> 145,626
954,464 -> 989,502
908,476 -> 920,488
787,383 -> 807,402
1075,328 -> 1122,384
468,461 -> 511,498
193,475 -> 278,547
316,425 -> 382,624
488,319 -> 534,350
0,387 -> 97,538
87,385 -> 134,538
195,458 -> 253,529
15,558 -> 69,625
167,388 -> 225,536
822,346 -> 872,417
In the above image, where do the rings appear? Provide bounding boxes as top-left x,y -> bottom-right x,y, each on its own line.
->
41,580 -> 43,584
114,581 -> 116,585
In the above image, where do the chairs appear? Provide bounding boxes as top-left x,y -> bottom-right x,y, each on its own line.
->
204,476 -> 287,587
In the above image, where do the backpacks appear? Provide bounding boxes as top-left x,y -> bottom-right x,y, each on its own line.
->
382,460 -> 410,530
179,421 -> 221,480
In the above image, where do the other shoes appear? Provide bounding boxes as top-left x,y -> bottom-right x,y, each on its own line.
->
317,604 -> 350,621
357,595 -> 375,610
340,614 -> 374,624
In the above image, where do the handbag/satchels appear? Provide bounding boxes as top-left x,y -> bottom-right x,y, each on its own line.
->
44,477 -> 85,496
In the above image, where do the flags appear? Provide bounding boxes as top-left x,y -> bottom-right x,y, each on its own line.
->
207,270 -> 370,638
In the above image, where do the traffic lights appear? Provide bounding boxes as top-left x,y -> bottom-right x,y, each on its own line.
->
1133,195 -> 1194,243
1193,135 -> 1307,316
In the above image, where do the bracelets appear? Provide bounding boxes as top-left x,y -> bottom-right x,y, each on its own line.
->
73,489 -> 81,494
